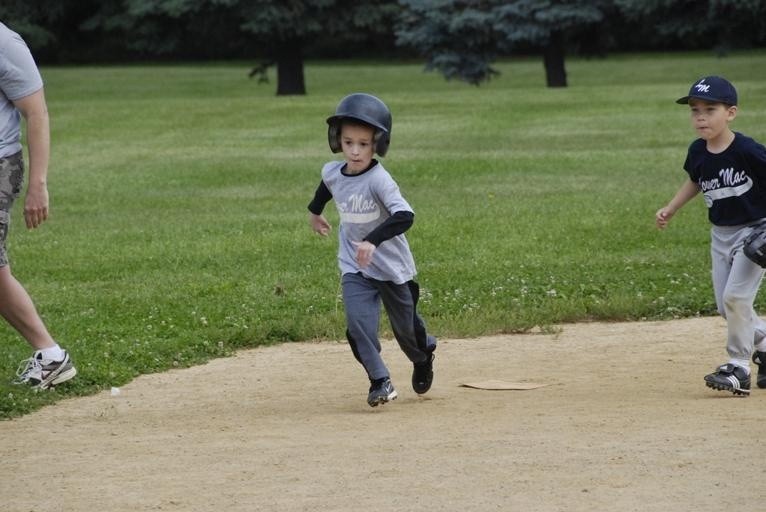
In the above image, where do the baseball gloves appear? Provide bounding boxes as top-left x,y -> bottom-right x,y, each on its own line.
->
743,221 -> 766,268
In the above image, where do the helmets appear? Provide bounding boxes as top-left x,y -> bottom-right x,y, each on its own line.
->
326,93 -> 391,156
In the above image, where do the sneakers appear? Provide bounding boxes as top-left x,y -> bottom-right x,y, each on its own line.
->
367,377 -> 397,406
752,351 -> 766,388
13,350 -> 77,392
412,352 -> 432,394
704,363 -> 751,395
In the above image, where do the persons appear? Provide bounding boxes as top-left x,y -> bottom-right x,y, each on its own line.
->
655,75 -> 766,397
0,22 -> 76,392
308,94 -> 436,407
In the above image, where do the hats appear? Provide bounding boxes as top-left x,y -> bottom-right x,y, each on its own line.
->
676,76 -> 737,106
